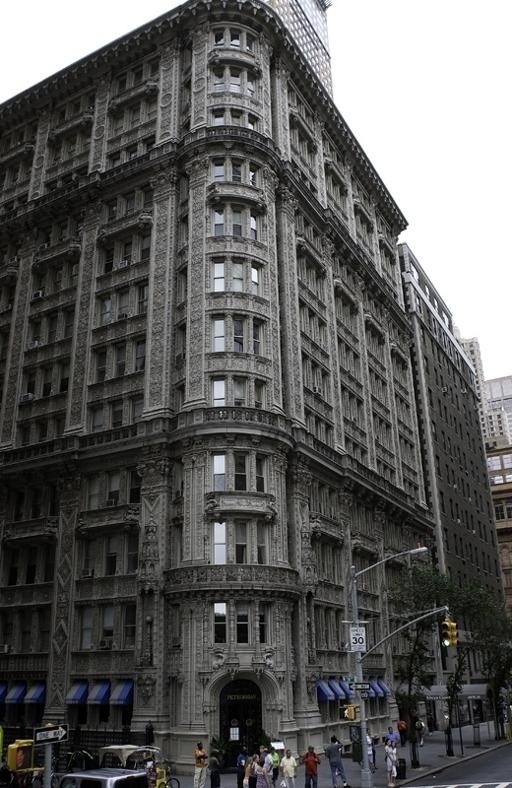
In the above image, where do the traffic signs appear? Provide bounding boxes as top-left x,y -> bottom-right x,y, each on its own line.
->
351,627 -> 366,652
348,683 -> 370,690
34,724 -> 69,747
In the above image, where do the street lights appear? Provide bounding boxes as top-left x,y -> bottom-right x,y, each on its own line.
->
349,547 -> 428,787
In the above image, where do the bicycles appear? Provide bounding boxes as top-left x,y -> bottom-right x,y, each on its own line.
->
159,769 -> 180,788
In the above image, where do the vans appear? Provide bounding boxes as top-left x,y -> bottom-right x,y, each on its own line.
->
58,767 -> 148,788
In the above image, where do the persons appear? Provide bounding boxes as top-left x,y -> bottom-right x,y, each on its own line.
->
417,715 -> 432,747
383,738 -> 396,787
235,742 -> 299,787
324,735 -> 352,788
391,740 -> 398,786
385,726 -> 400,749
366,727 -> 373,774
300,744 -> 322,787
333,733 -> 342,775
397,715 -> 407,748
368,732 -> 380,770
208,740 -> 221,788
194,740 -> 210,788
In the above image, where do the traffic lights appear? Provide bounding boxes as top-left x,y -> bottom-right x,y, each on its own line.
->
6,739 -> 35,774
443,620 -> 459,648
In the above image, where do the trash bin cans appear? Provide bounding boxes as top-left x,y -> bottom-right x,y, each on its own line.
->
396,759 -> 406,779
